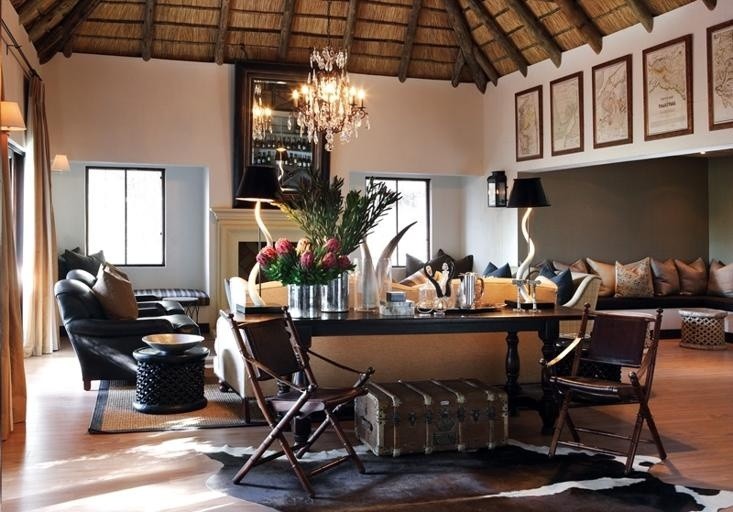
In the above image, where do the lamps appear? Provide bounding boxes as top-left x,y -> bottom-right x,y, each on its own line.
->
235,165 -> 281,307
0,101 -> 27,131
275,147 -> 289,183
288,1 -> 372,152
50,153 -> 71,172
253,85 -> 272,141
506,175 -> 552,303
487,170 -> 507,207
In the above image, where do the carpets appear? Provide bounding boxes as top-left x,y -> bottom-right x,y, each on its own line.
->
194,438 -> 733,512
88,368 -> 653,435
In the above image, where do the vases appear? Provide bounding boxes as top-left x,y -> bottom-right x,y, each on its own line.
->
287,285 -> 317,321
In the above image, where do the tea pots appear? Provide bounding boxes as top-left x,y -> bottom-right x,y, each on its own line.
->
456,272 -> 484,309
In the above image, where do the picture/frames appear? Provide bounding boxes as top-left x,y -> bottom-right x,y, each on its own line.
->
515,84 -> 543,162
705,20 -> 733,132
641,33 -> 694,142
591,54 -> 633,149
549,71 -> 585,156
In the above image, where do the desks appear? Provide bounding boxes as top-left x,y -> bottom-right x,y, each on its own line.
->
212,305 -> 597,425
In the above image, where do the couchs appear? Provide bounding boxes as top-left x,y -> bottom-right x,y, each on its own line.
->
211,273 -> 558,394
596,295 -> 733,333
505,264 -> 603,352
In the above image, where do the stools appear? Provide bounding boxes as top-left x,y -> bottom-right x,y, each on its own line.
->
678,307 -> 728,351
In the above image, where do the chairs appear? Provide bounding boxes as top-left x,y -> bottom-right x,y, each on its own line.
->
546,302 -> 667,476
218,305 -> 376,499
52,247 -> 209,390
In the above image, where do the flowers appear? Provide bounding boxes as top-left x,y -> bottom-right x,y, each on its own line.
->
254,237 -> 356,285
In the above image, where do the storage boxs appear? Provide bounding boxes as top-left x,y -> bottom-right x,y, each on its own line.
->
354,377 -> 509,457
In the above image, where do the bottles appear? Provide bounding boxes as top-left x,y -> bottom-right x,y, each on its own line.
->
254,135 -> 312,169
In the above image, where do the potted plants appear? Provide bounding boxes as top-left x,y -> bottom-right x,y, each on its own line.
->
270,166 -> 403,313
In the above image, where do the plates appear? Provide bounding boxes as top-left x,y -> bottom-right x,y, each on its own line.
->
416,302 -> 508,310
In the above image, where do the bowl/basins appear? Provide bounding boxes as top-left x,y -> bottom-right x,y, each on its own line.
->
142,333 -> 205,354
